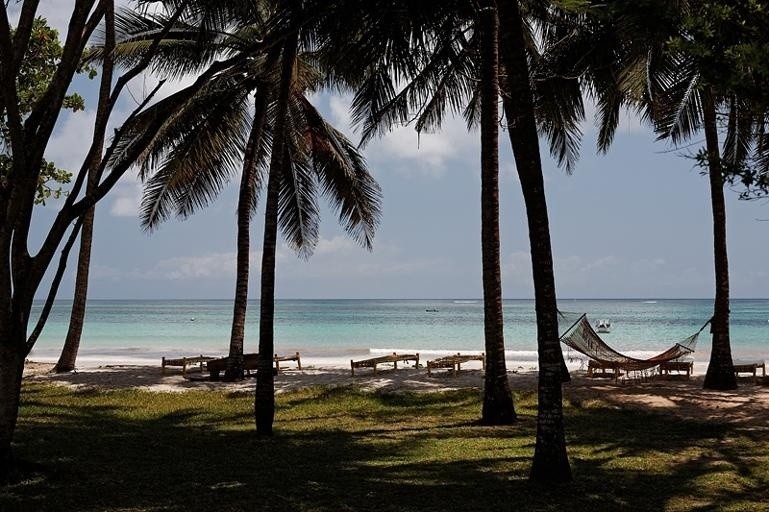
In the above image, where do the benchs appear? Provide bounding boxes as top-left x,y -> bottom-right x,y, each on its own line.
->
427,350 -> 486,377
586,354 -> 693,381
350,351 -> 420,377
272,350 -> 302,368
732,357 -> 765,378
164,348 -> 217,374
